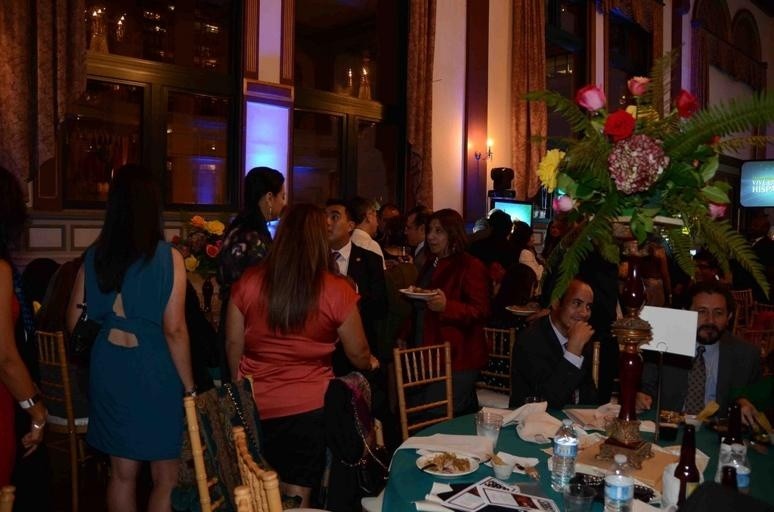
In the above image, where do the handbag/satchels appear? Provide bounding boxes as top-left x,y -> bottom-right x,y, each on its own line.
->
70,304 -> 98,361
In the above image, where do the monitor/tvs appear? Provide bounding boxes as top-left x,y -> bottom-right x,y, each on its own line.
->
489,198 -> 532,226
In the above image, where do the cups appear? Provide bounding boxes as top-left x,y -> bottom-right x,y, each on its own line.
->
561,483 -> 597,511
475,413 -> 503,448
492,459 -> 515,480
397,246 -> 411,262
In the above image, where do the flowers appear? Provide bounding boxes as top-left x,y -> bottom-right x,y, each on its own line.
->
168,209 -> 229,276
514,30 -> 773,309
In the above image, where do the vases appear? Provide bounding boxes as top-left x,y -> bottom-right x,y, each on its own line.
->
589,210 -> 691,471
200,271 -> 216,318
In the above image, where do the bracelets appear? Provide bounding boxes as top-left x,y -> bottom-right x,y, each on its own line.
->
183,383 -> 199,397
16,391 -> 43,411
29,421 -> 47,432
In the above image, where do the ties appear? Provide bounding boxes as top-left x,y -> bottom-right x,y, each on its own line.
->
686,346 -> 705,415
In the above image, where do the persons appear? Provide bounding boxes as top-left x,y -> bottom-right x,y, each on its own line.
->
344,198 -> 388,270
541,221 -> 560,259
401,207 -> 487,418
510,277 -> 611,420
224,205 -> 380,508
466,211 -> 544,326
1,162 -> 51,511
316,199 -> 387,420
37,262 -> 89,421
538,208 -> 619,323
376,203 -> 401,242
397,205 -> 434,271
748,217 -> 774,268
201,168 -> 286,392
64,161 -> 195,511
634,284 -> 762,427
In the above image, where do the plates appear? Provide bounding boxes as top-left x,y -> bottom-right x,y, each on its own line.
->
398,288 -> 438,299
505,305 -> 540,316
484,451 -> 540,476
415,452 -> 479,480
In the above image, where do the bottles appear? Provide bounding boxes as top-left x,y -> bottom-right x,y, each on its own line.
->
721,464 -> 738,492
728,443 -> 752,495
550,419 -> 578,493
603,453 -> 634,512
714,402 -> 748,484
673,425 -> 700,509
